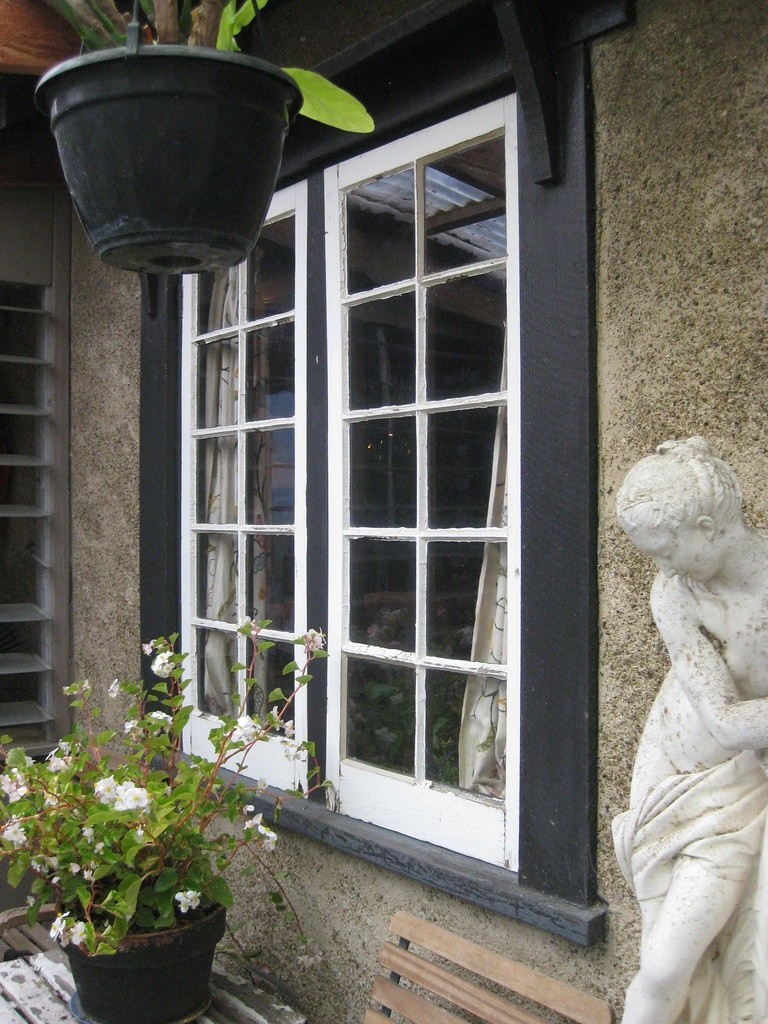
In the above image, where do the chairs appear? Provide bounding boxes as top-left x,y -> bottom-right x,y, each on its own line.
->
363,910 -> 618,1024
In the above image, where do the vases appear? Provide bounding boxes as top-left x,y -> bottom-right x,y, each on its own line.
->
51,902 -> 229,1024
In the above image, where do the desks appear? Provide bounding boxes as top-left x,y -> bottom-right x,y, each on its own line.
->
0,945 -> 306,1024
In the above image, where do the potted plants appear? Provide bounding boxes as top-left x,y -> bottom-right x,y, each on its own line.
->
31,0 -> 379,276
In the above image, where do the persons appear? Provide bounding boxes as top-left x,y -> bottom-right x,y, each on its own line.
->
612,433 -> 768,1024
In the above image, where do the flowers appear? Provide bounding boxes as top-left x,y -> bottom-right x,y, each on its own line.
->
345,603 -> 475,784
0,616 -> 345,958
